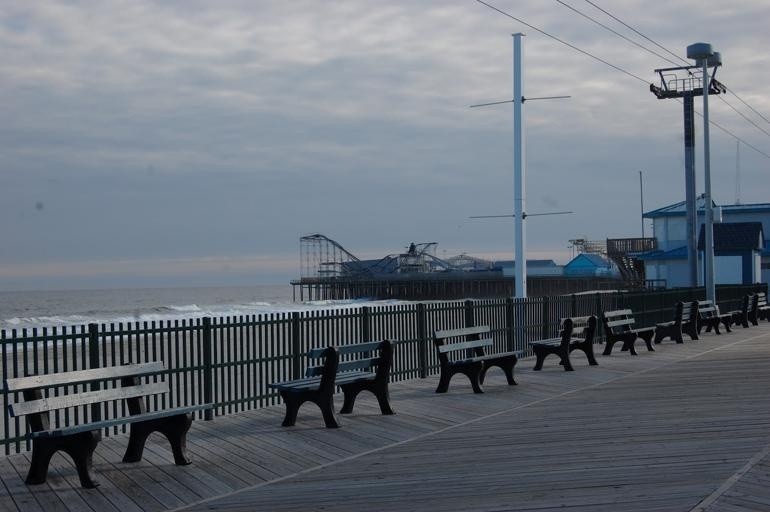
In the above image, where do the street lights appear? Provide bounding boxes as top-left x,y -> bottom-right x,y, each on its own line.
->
686,42 -> 722,305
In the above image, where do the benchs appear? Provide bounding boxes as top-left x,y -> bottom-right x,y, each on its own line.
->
7,360 -> 212,490
267,339 -> 394,429
530,292 -> 769,371
433,326 -> 524,393
